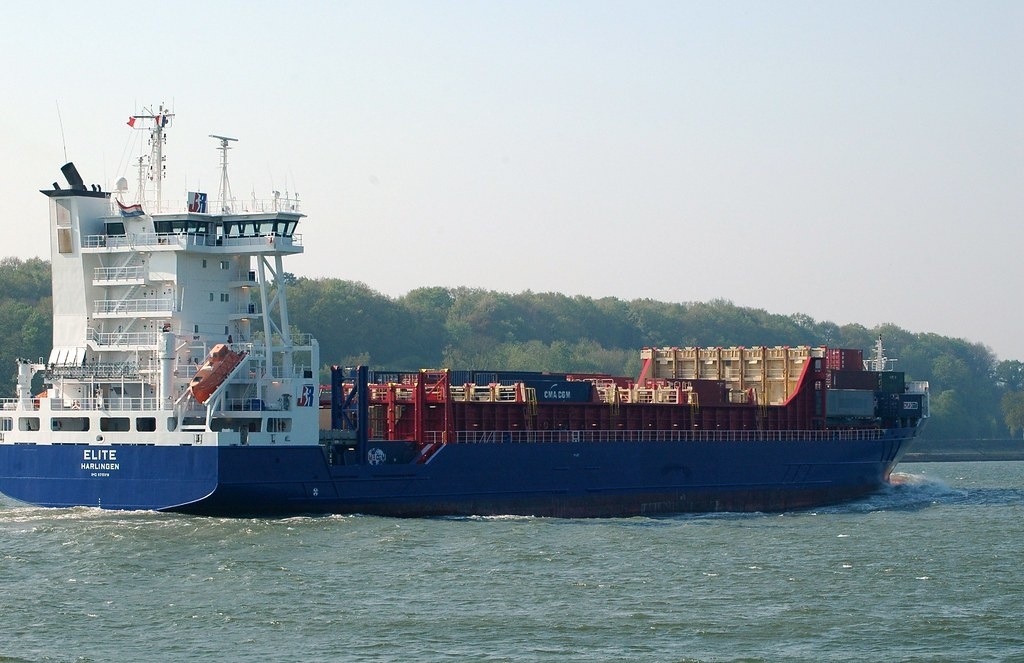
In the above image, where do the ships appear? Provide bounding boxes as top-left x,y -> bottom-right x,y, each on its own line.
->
0,97 -> 931,517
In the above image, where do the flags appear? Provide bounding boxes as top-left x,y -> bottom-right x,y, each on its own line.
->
155,115 -> 167,127
127,117 -> 136,127
115,198 -> 146,217
